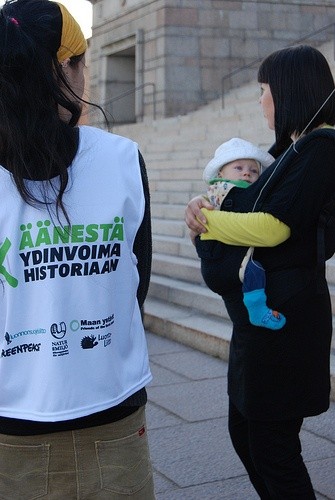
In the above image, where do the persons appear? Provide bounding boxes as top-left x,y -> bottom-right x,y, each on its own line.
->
184,44 -> 335,500
0,0 -> 155,500
195,137 -> 286,330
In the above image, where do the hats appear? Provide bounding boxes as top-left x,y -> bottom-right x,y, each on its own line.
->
202,138 -> 276,187
